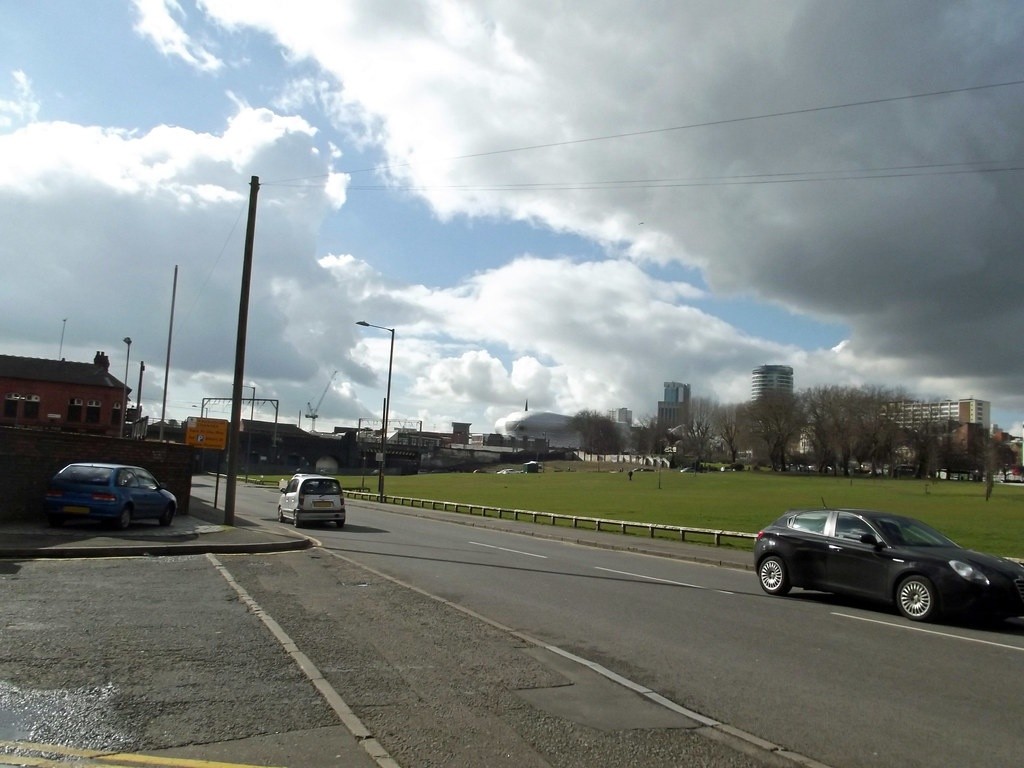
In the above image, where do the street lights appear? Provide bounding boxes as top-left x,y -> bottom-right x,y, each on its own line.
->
192,404 -> 208,474
354,320 -> 396,502
119,336 -> 133,438
232,384 -> 255,483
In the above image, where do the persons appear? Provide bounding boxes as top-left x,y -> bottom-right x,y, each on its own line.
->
628,470 -> 633,480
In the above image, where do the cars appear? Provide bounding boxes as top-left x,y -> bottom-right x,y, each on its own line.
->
45,463 -> 178,531
753,507 -> 1024,630
277,473 -> 345,529
721,463 -> 745,471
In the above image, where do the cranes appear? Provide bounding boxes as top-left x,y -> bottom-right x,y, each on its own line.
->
304,371 -> 337,432
